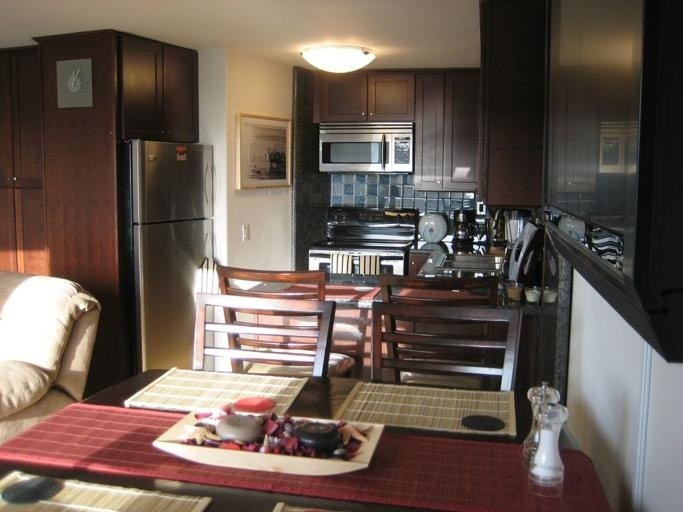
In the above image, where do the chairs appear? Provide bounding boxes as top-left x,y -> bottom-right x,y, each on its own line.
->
193,293 -> 335,378
214,260 -> 355,377
379,273 -> 499,389
372,302 -> 523,391
0,271 -> 101,447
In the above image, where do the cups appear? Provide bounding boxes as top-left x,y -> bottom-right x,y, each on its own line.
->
543,286 -> 558,305
505,282 -> 542,305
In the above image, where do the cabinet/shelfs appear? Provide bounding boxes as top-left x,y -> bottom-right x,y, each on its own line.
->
321,69 -> 415,121
415,68 -> 481,192
0,29 -> 199,399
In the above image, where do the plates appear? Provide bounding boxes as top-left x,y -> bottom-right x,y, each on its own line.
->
152,409 -> 386,477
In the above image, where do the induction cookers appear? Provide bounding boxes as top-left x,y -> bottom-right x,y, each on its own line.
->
309,206 -> 419,253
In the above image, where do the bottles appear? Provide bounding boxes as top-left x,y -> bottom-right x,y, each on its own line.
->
522,382 -> 569,497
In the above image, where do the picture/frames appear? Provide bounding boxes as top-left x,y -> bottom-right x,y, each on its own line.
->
235,113 -> 292,191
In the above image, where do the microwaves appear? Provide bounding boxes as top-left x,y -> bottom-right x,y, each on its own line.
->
316,122 -> 416,174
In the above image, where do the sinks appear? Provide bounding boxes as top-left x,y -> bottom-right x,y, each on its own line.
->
440,253 -> 505,264
432,261 -> 505,271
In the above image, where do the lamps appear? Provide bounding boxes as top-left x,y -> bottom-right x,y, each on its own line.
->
301,45 -> 376,73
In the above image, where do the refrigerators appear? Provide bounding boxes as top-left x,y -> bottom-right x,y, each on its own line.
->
118,137 -> 214,375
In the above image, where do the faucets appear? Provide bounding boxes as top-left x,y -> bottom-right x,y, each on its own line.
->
492,208 -> 512,245
